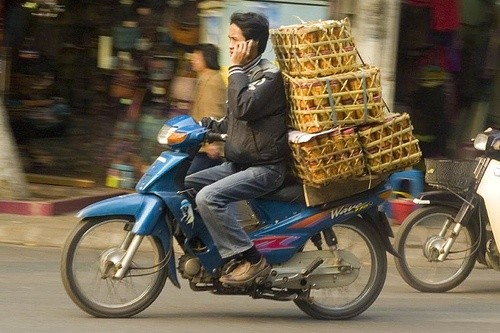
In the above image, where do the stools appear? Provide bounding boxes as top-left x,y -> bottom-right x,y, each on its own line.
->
384,170 -> 424,218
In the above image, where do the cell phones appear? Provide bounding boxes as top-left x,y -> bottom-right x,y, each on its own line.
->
242,41 -> 249,49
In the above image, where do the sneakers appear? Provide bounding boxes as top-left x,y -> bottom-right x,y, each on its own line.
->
220,257 -> 270,286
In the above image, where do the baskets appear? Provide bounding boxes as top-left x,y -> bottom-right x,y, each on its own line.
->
425,159 -> 479,192
357,114 -> 422,177
288,128 -> 366,189
280,68 -> 384,135
269,17 -> 358,76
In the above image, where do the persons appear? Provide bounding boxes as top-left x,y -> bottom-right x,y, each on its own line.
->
186,43 -> 227,176
185,13 -> 284,287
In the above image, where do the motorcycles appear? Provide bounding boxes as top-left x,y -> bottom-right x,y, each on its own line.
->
392,126 -> 500,293
60,112 -> 403,318
